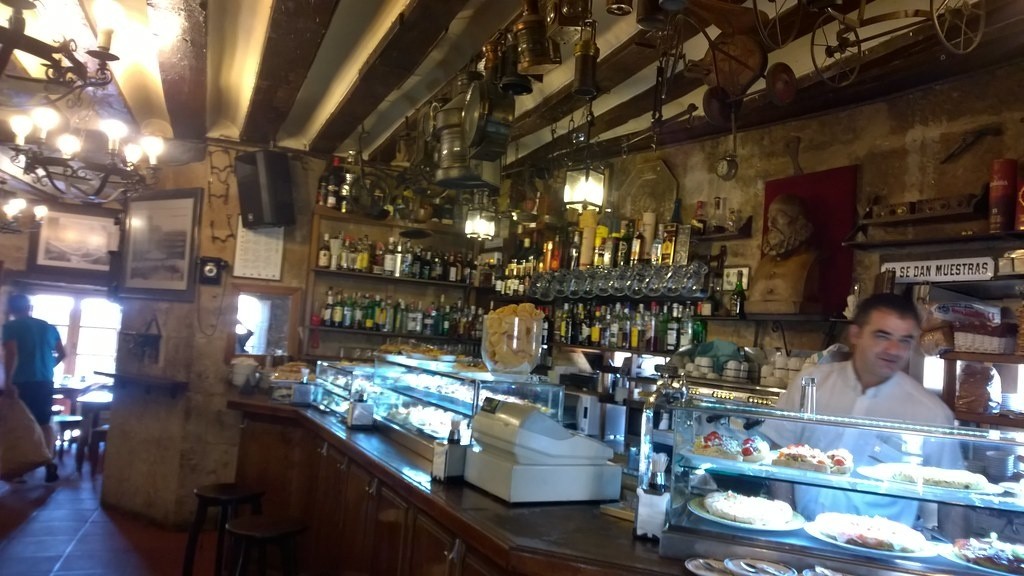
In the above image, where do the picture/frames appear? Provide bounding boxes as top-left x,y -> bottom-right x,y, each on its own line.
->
117,186 -> 204,303
26,202 -> 124,287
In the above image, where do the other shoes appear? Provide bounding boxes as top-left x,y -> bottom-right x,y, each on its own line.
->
44,475 -> 61,485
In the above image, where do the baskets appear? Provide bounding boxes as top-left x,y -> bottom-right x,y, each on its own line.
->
953,332 -> 1016,354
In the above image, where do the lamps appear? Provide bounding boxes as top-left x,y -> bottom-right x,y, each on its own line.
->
564,85 -> 605,215
464,185 -> 497,241
0,0 -> 165,234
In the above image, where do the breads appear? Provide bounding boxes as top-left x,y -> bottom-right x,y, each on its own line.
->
956,363 -> 1001,414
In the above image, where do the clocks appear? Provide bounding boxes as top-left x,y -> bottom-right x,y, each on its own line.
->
716,157 -> 738,180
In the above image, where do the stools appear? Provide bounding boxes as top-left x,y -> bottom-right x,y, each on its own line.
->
52,415 -> 82,462
226,515 -> 307,576
88,424 -> 110,478
182,482 -> 267,576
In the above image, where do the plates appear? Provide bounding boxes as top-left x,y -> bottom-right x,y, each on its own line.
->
724,557 -> 799,576
802,569 -> 853,576
856,466 -> 1005,496
961,393 -> 1019,478
934,541 -> 1024,575
684,557 -> 735,576
803,519 -> 939,558
687,494 -> 807,531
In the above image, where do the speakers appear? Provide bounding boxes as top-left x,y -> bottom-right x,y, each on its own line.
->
235,150 -> 296,230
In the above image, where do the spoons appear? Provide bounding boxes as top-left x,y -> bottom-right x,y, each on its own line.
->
699,559 -> 733,575
739,561 -> 786,576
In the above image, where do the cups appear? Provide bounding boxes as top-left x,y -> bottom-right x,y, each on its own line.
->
683,356 -> 813,389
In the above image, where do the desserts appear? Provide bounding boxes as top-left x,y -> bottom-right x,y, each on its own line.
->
690,431 -> 1024,574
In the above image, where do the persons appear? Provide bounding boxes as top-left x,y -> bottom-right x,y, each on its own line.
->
235,320 -> 252,352
760,293 -> 962,538
745,195 -> 820,301
2,295 -> 66,484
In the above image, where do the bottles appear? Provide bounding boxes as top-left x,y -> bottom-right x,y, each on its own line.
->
309,156 -> 745,352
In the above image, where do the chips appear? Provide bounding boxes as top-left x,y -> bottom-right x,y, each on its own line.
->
485,302 -> 544,370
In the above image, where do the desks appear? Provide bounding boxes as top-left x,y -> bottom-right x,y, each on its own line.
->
74,388 -> 114,474
52,380 -> 94,448
225,388 -> 699,575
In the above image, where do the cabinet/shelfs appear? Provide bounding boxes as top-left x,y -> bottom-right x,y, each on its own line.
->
300,205 -> 1024,575
